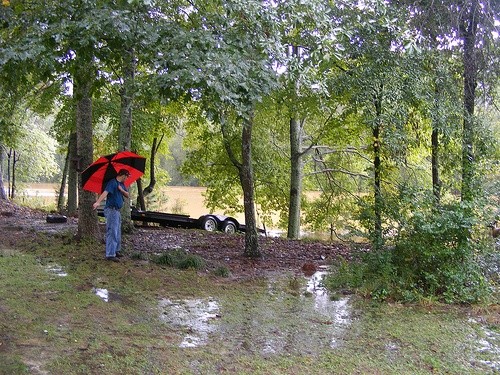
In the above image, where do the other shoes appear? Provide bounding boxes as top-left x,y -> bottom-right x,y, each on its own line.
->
107,257 -> 120,262
115,252 -> 124,257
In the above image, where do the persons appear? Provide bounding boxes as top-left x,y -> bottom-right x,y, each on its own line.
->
92,169 -> 130,262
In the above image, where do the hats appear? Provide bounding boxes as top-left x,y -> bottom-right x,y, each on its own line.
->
119,170 -> 131,177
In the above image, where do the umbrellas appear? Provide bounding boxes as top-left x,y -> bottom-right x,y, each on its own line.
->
81,152 -> 146,202
